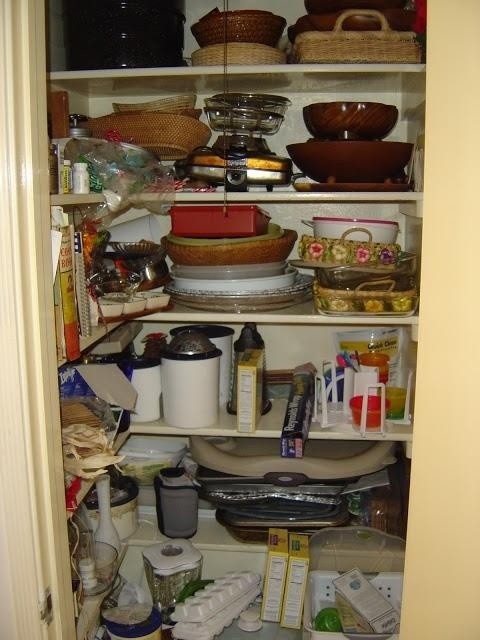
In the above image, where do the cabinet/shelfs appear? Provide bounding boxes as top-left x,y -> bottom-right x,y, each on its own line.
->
42,0 -> 427,624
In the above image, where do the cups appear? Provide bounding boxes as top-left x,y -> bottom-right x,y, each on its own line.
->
358,352 -> 390,384
377,386 -> 408,420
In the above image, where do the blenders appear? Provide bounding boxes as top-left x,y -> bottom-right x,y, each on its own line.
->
141,537 -> 204,622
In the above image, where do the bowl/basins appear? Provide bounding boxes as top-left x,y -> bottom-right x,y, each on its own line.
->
285,141 -> 415,182
302,101 -> 399,140
87,269 -> 143,301
349,394 -> 392,427
108,241 -> 167,257
83,540 -> 119,597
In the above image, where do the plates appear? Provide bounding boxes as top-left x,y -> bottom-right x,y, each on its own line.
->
164,272 -> 315,312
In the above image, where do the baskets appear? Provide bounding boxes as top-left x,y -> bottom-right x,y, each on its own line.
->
80,95 -> 211,161
297,228 -> 400,270
191,10 -> 287,48
290,9 -> 422,63
161,230 -> 298,266
191,42 -> 287,66
312,276 -> 420,318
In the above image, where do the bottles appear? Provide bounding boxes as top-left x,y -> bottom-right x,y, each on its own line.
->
48,143 -> 90,194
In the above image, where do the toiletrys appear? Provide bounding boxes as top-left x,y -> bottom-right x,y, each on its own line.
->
307,607 -> 342,632
